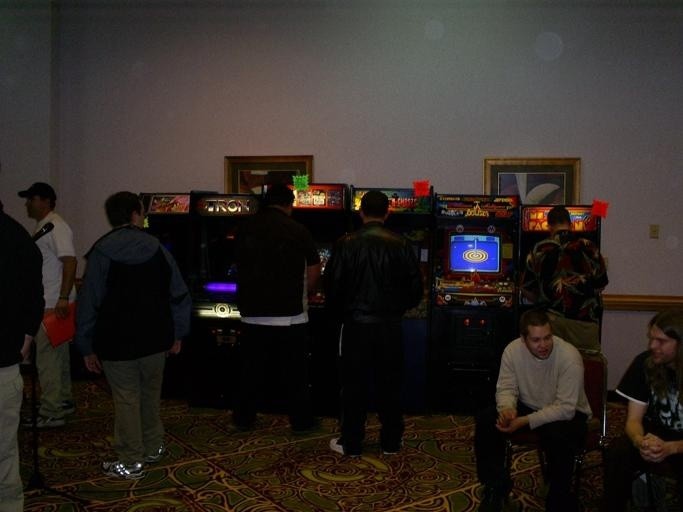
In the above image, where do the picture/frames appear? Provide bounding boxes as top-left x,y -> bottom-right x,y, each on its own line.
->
219,152 -> 314,194
480,153 -> 585,206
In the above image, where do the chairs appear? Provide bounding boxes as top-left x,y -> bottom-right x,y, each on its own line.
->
503,347 -> 609,511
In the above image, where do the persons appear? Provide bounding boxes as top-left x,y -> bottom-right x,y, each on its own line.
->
77,190 -> 193,479
227,181 -> 323,435
321,189 -> 425,456
520,204 -> 610,354
0,202 -> 46,511
599,306 -> 683,512
473,305 -> 593,512
16,181 -> 79,428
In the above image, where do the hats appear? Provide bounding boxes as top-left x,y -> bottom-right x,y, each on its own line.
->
18,182 -> 55,197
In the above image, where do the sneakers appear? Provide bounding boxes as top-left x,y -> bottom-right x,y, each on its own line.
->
102,444 -> 163,480
330,438 -> 359,458
21,401 -> 75,428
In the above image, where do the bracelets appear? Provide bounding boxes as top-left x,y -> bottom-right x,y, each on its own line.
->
58,295 -> 69,301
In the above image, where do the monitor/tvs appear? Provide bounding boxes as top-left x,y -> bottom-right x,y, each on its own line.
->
450,232 -> 500,273
202,252 -> 241,303
305,245 -> 336,308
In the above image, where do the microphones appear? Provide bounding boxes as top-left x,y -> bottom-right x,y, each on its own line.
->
31,221 -> 54,242
